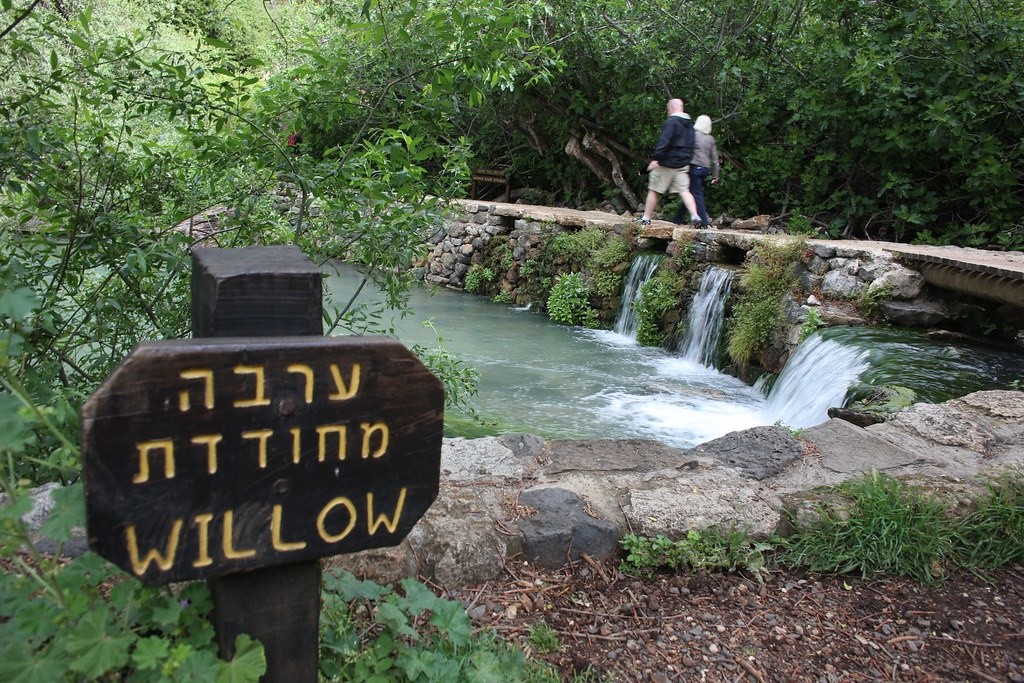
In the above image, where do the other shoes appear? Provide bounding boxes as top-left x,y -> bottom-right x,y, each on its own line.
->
668,218 -> 680,225
688,218 -> 703,229
702,224 -> 711,230
632,215 -> 650,224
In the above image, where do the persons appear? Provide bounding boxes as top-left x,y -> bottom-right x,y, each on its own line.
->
289,129 -> 303,160
667,115 -> 719,229
632,98 -> 703,229
